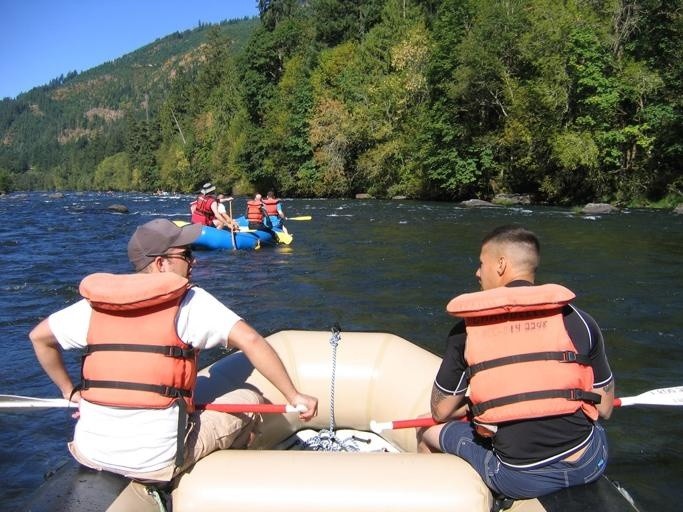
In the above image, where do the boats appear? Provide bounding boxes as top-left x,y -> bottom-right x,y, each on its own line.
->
4,326 -> 641,512
172,214 -> 283,253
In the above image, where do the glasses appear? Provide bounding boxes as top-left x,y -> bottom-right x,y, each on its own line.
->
144,248 -> 194,264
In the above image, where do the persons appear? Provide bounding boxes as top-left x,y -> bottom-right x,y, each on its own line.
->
262,191 -> 288,234
28,219 -> 318,490
191,182 -> 234,230
415,224 -> 615,500
245,194 -> 278,247
215,193 -> 232,224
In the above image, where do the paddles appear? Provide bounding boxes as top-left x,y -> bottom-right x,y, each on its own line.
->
370,385 -> 682,434
286,215 -> 312,220
0,395 -> 309,414
276,231 -> 292,245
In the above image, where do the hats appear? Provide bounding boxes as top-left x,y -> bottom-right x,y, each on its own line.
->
200,182 -> 217,195
126,216 -> 203,273
